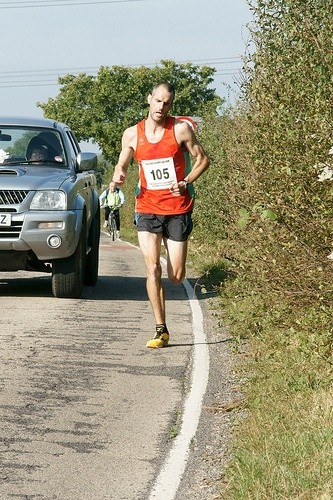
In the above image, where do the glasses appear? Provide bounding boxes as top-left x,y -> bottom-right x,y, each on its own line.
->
32,152 -> 46,157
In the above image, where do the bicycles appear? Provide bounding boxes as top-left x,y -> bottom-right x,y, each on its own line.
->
103,205 -> 121,241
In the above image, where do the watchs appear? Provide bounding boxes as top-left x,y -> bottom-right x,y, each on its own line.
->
181,179 -> 190,188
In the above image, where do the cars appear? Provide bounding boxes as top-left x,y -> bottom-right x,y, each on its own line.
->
0,113 -> 101,298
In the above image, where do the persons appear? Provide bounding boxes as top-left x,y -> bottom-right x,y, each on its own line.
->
27,146 -> 63,164
112,81 -> 212,350
100,181 -> 125,240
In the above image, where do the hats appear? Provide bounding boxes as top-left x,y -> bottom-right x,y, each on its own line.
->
26,136 -> 65,165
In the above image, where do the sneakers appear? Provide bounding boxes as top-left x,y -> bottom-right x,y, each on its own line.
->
104,221 -> 108,228
146,324 -> 169,347
116,231 -> 121,237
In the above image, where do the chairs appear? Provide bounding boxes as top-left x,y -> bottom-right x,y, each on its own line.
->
26,134 -> 59,164
